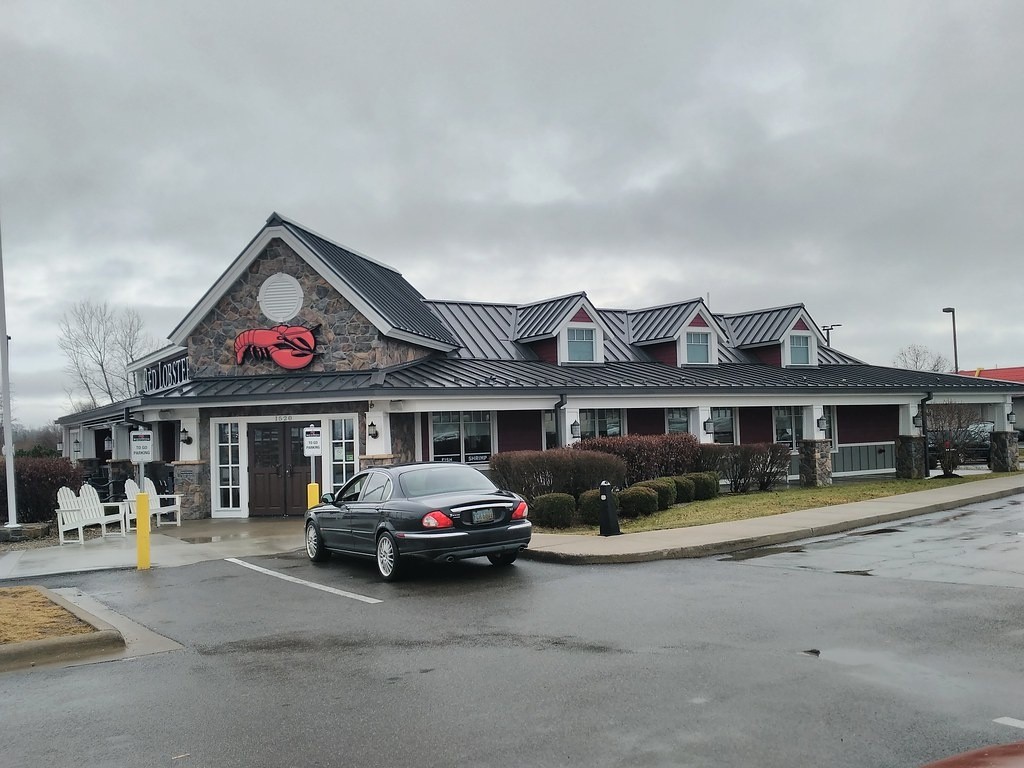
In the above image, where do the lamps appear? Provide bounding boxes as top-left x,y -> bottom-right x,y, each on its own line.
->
74,438 -> 80,452
913,412 -> 922,427
704,417 -> 714,434
104,435 -> 114,452
1007,411 -> 1016,424
56,439 -> 63,451
368,421 -> 379,439
571,420 -> 581,438
816,415 -> 827,431
179,428 -> 192,445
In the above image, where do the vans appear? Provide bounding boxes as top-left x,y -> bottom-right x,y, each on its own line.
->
928,429 -> 991,465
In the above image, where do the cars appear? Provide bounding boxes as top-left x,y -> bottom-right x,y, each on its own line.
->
304,463 -> 532,582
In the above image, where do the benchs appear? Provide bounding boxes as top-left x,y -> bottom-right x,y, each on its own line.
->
123,476 -> 184,533
55,483 -> 130,546
